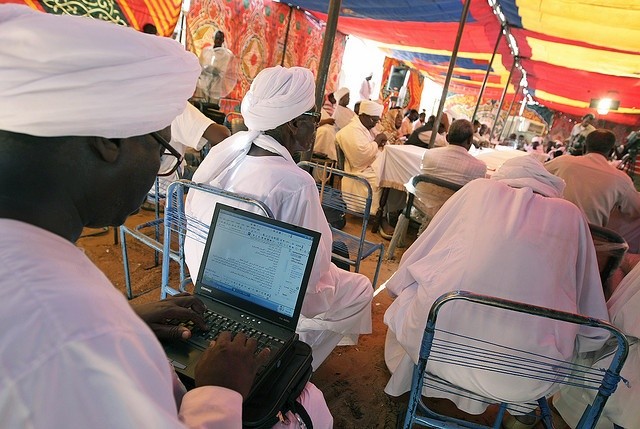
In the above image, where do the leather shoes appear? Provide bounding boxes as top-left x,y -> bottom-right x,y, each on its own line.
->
503,406 -> 543,429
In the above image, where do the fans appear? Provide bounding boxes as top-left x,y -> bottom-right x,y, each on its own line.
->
199,46 -> 239,102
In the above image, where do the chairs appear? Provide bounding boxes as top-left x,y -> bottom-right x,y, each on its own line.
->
159,178 -> 275,300
118,167 -> 186,298
395,291 -> 630,429
334,140 -> 345,180
296,161 -> 383,289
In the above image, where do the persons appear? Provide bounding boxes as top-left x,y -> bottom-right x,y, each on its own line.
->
331,87 -> 357,129
406,112 -> 450,150
503,133 -> 568,161
375,107 -> 402,145
150,100 -> 232,197
605,251 -> 640,429
403,109 -> 429,137
0,4 -> 335,429
545,128 -> 640,229
322,93 -> 336,117
357,72 -> 372,100
198,30 -> 234,103
313,90 -> 336,161
384,154 -> 611,429
335,100 -> 405,235
183,66 -> 373,334
472,116 -> 498,149
410,120 -> 486,222
568,114 -> 596,155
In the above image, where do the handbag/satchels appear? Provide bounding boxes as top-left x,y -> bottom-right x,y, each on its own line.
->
317,183 -> 346,230
243,339 -> 314,429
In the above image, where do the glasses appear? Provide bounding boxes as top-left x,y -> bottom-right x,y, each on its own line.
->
150,131 -> 183,176
368,116 -> 380,124
303,112 -> 321,123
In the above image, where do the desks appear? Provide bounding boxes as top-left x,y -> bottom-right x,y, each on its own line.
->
371,145 -> 537,234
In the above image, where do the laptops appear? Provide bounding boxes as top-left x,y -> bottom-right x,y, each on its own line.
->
160,201 -> 323,407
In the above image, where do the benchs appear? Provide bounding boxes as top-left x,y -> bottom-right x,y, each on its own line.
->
385,176 -> 628,295
